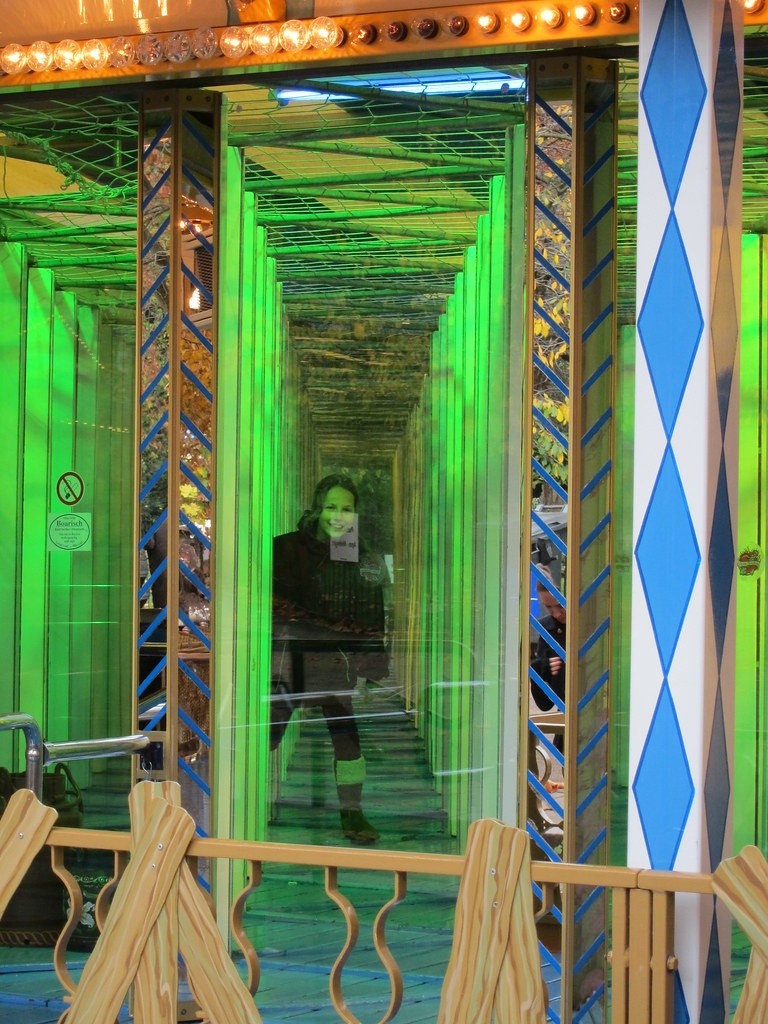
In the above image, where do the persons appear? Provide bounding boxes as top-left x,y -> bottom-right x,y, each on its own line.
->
271,476 -> 391,844
531,579 -> 566,756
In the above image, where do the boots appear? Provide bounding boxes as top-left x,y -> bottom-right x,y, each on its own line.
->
333,754 -> 380,844
